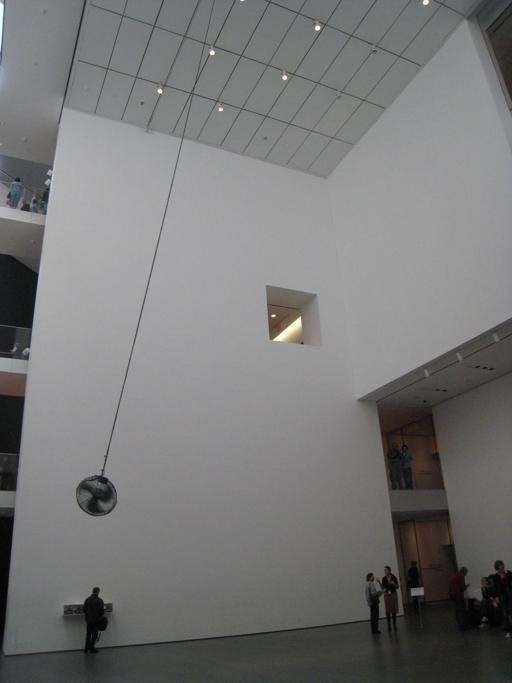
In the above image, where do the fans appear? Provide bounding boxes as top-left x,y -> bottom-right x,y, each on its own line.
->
76,475 -> 117,516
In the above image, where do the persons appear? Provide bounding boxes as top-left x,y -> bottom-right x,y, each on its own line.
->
9,176 -> 24,209
386,441 -> 406,490
7,342 -> 23,360
40,187 -> 49,215
477,576 -> 499,629
365,572 -> 385,635
376,566 -> 400,632
399,444 -> 416,490
448,566 -> 470,600
406,559 -> 421,610
1,453 -> 13,490
494,559 -> 512,639
30,189 -> 42,213
82,586 -> 105,654
